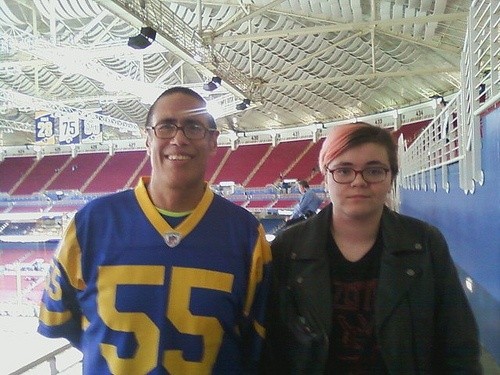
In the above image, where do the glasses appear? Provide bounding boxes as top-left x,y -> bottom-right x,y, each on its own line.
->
145,120 -> 218,140
325,166 -> 391,184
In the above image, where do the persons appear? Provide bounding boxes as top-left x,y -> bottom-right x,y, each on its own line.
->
36,85 -> 274,374
211,173 -> 286,200
266,121 -> 487,375
273,179 -> 321,236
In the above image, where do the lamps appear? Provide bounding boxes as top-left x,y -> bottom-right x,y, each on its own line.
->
235,98 -> 251,110
126,27 -> 158,50
204,76 -> 221,91
